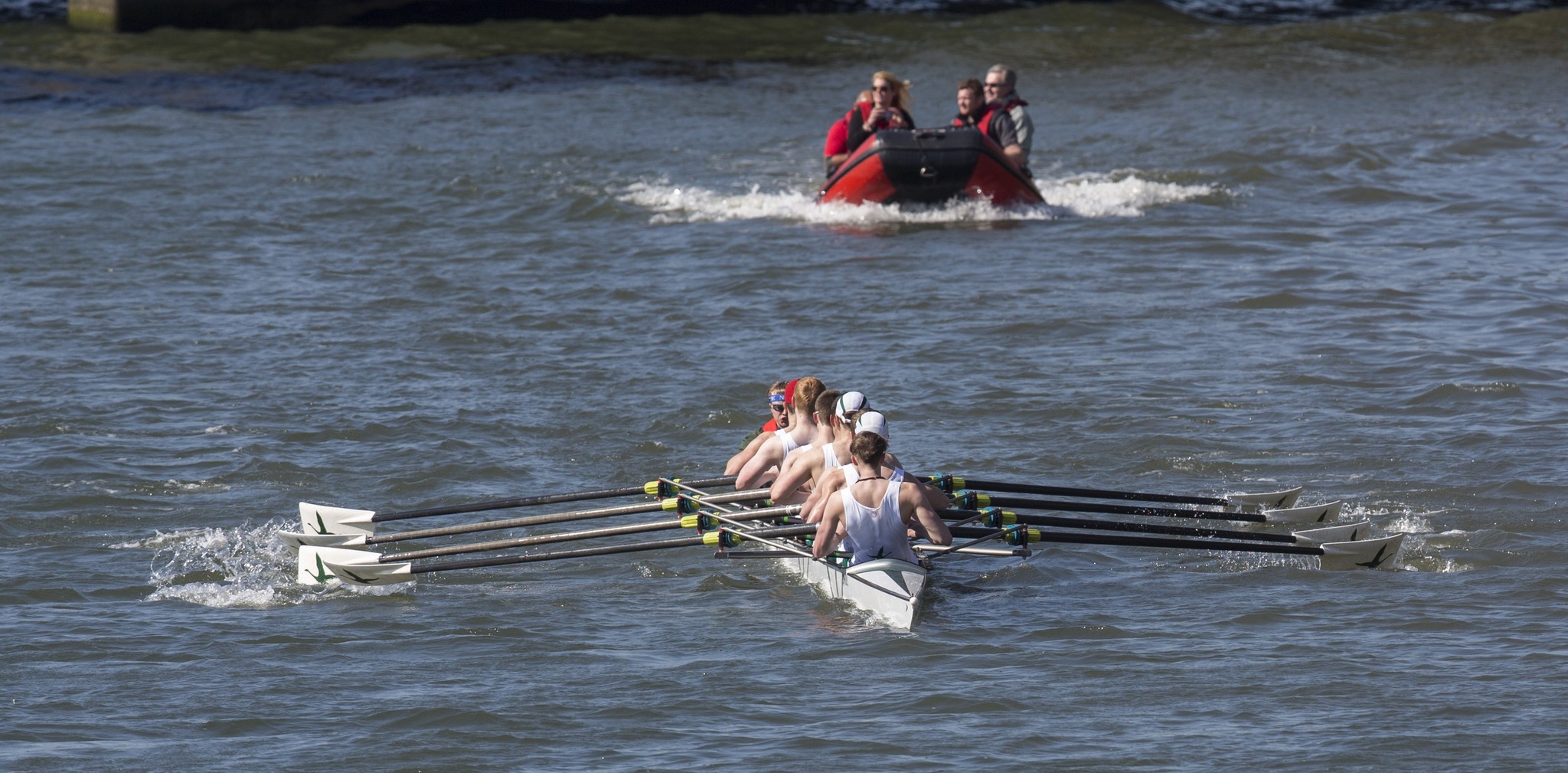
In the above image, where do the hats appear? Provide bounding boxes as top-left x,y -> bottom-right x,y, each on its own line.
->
784,380 -> 799,404
855,411 -> 890,441
836,391 -> 871,423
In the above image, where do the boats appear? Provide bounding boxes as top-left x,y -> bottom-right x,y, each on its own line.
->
815,124 -> 1047,208
754,482 -> 928,631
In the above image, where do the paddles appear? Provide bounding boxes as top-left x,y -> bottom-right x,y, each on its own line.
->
912,474 -> 1303,515
277,486 -> 770,549
946,491 -> 1344,527
948,524 -> 1405,571
297,474 -> 772,537
296,502 -> 805,589
322,521 -> 823,588
936,509 -> 1371,546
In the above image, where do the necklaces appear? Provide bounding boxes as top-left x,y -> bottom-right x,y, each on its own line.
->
854,476 -> 886,482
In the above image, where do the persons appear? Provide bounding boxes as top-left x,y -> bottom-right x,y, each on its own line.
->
848,71 -> 916,154
724,376 -> 953,567
824,90 -> 873,181
951,79 -> 1023,168
983,64 -> 1035,179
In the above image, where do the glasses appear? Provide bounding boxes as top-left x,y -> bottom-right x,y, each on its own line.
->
770,403 -> 784,413
872,87 -> 893,92
984,81 -> 1009,88
